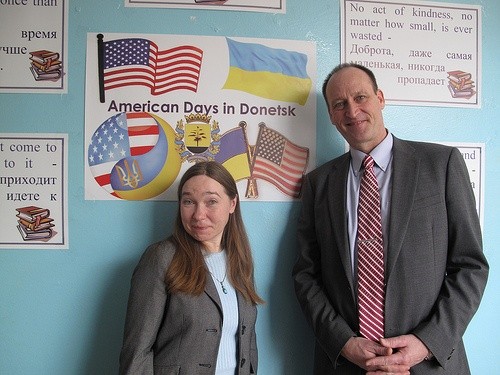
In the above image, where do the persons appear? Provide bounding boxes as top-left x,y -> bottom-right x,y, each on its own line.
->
118,161 -> 265,375
290,64 -> 489,375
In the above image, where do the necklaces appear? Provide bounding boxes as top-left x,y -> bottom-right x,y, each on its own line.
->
211,262 -> 229,296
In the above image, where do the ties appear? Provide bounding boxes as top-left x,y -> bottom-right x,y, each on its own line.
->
356,156 -> 385,341
